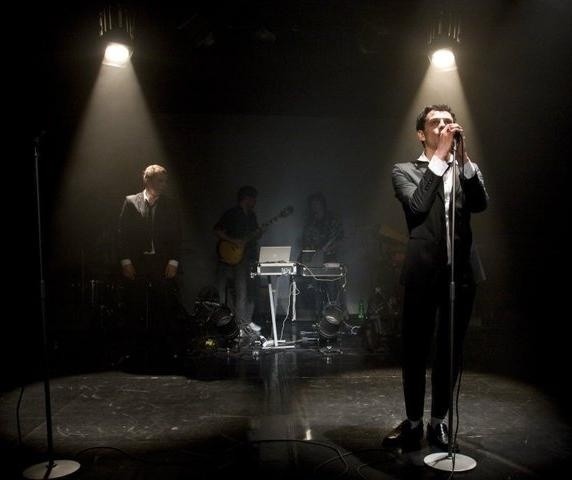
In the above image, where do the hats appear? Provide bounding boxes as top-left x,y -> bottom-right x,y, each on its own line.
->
236,186 -> 257,204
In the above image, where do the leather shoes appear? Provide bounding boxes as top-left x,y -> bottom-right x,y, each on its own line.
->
426,422 -> 459,450
382,419 -> 424,449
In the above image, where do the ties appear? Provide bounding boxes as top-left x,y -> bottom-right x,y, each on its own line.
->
145,199 -> 156,252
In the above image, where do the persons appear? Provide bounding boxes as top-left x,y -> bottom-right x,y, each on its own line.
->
118,164 -> 182,308
297,195 -> 346,313
381,102 -> 488,452
213,186 -> 262,323
